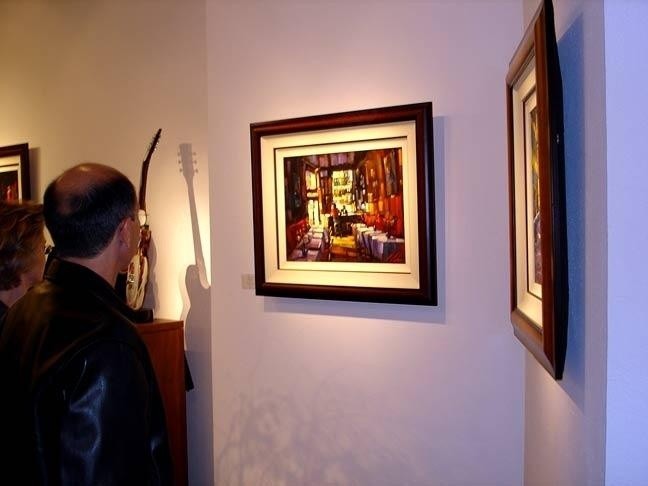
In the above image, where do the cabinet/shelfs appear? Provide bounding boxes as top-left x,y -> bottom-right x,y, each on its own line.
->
135,320 -> 189,486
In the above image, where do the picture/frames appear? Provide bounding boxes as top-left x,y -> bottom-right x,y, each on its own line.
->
505,1 -> 569,382
250,99 -> 439,311
1,142 -> 31,207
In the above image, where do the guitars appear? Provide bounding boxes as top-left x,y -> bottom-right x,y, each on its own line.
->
177,142 -> 210,398
114,128 -> 162,311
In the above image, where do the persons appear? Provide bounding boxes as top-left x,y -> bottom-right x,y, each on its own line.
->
0,199 -> 47,317
5,184 -> 12,201
0,163 -> 174,485
331,204 -> 342,233
